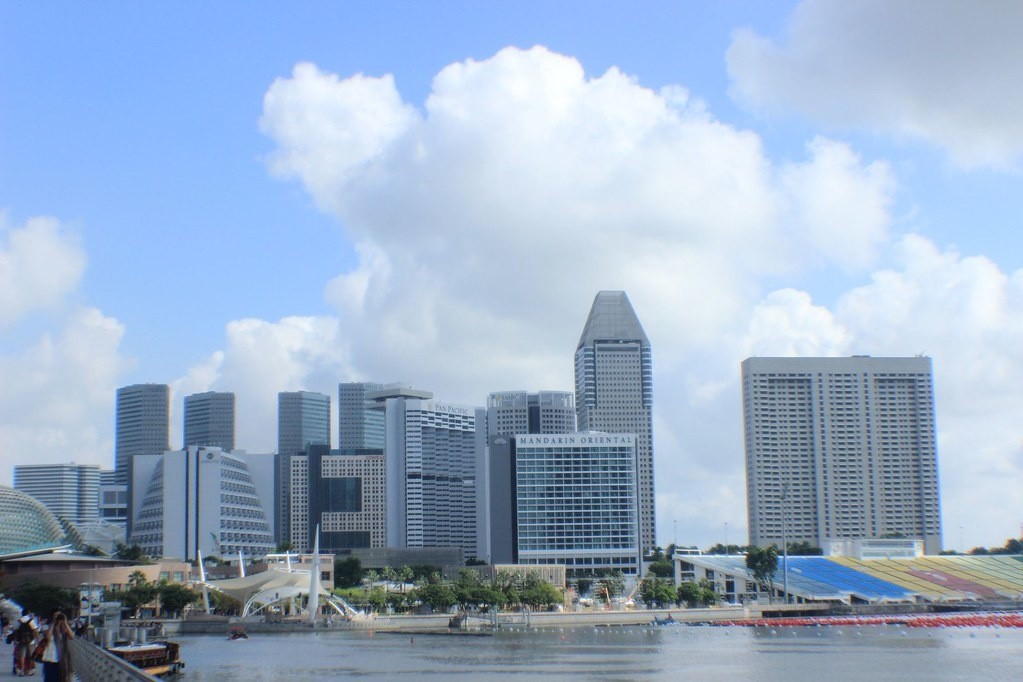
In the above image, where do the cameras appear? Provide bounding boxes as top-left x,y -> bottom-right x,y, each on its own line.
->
56,614 -> 65,619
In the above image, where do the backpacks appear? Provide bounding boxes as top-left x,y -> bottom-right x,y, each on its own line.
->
14,618 -> 35,646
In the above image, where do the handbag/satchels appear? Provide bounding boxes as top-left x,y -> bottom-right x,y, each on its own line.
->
30,638 -> 48,662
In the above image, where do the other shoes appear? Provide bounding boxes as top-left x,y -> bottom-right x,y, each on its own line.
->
26,668 -> 35,675
16,667 -> 24,676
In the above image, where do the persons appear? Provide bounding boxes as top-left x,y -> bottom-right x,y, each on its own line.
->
76,617 -> 89,639
14,609 -> 41,676
42,608 -> 74,682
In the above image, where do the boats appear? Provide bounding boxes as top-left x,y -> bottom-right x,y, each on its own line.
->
652,612 -> 675,625
106,641 -> 185,676
226,626 -> 247,640
